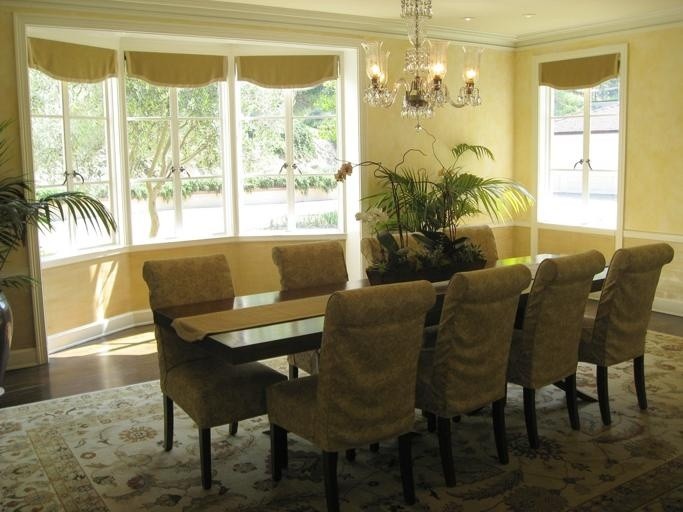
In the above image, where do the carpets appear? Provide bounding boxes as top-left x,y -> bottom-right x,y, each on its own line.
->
1,315 -> 683,512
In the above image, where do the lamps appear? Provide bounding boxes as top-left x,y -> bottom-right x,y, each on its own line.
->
360,0 -> 483,132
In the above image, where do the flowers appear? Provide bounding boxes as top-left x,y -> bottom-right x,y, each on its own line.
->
333,143 -> 536,277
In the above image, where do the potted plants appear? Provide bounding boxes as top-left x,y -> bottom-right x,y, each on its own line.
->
0,119 -> 119,398
